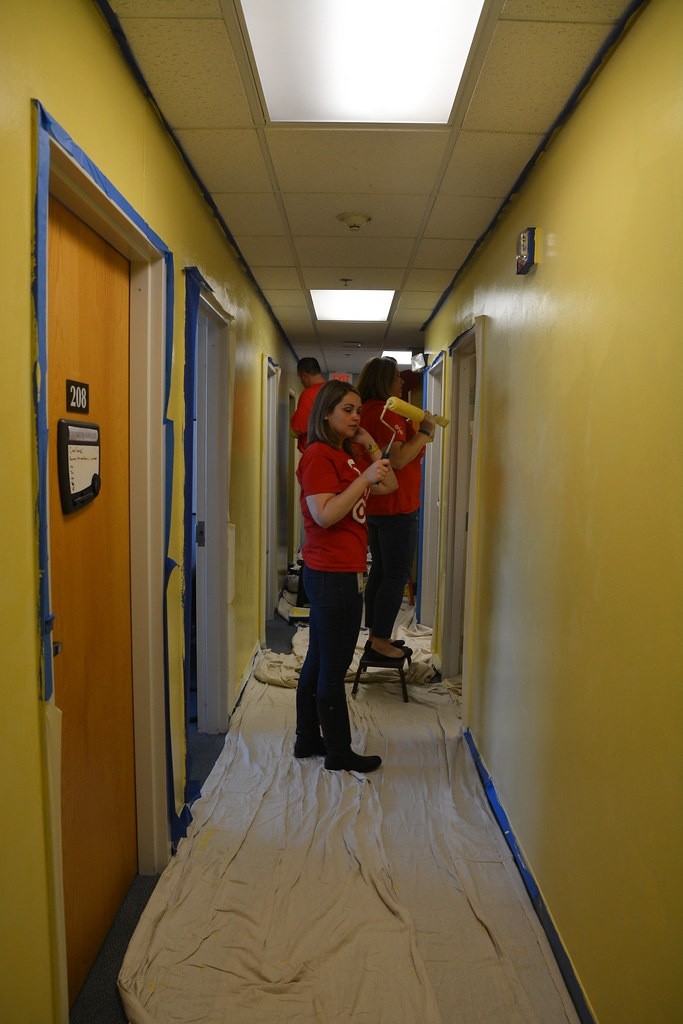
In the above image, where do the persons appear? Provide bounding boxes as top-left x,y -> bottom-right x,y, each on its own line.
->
354,356 -> 434,666
294,380 -> 398,775
290,357 -> 329,561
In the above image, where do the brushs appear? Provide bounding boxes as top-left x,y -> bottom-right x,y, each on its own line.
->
373,397 -> 425,485
432,414 -> 450,427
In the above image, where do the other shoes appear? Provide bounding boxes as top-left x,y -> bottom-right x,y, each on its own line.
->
324,750 -> 382,773
364,640 -> 413,661
293,736 -> 327,759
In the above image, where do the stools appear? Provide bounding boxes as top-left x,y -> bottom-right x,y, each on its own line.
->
351,646 -> 412,703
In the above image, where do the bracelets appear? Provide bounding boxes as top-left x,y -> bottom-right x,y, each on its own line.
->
418,429 -> 430,436
366,443 -> 378,454
